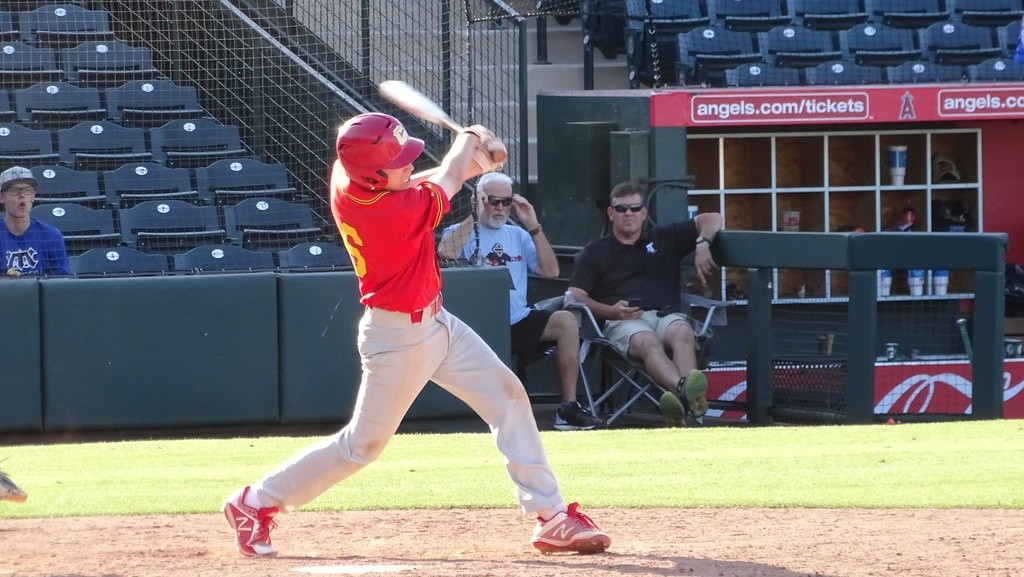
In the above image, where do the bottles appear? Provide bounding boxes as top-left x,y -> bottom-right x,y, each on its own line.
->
932,270 -> 950,293
889,146 -> 907,184
877,270 -> 892,295
835,225 -> 864,232
907,269 -> 926,295
901,197 -> 915,232
687,205 -> 698,219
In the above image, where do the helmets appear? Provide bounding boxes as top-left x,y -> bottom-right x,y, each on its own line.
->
336,113 -> 425,191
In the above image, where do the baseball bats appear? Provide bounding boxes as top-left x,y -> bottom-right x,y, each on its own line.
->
377,79 -> 507,164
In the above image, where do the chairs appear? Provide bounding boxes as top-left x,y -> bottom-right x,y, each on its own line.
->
534,253 -> 737,427
487,0 -> 1024,90
0,0 -> 357,277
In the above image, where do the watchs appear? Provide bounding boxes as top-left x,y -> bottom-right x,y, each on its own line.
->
696,236 -> 712,248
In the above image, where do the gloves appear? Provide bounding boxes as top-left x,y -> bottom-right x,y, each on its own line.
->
472,138 -> 508,175
461,125 -> 495,148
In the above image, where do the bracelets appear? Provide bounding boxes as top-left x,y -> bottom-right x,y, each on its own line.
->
529,224 -> 543,236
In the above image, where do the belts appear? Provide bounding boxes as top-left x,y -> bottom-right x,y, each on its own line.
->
411,293 -> 442,324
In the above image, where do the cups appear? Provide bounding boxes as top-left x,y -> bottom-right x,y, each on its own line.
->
885,342 -> 900,361
780,211 -> 800,231
1004,338 -> 1022,357
816,334 -> 834,356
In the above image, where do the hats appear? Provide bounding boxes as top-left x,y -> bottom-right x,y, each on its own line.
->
0,166 -> 37,191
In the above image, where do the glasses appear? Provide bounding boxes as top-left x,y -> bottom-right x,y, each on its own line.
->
611,204 -> 644,212
2,186 -> 34,196
483,196 -> 513,206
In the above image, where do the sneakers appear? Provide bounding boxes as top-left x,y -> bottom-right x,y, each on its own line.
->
223,486 -> 278,557
0,467 -> 28,502
677,369 -> 708,418
659,391 -> 688,427
553,401 -> 607,430
532,502 -> 611,554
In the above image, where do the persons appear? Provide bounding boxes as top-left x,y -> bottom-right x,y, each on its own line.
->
437,172 -> 607,430
565,182 -> 722,428
224,113 -> 610,558
0,165 -> 69,276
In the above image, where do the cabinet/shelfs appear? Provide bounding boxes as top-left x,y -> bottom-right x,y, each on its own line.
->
685,128 -> 983,306
